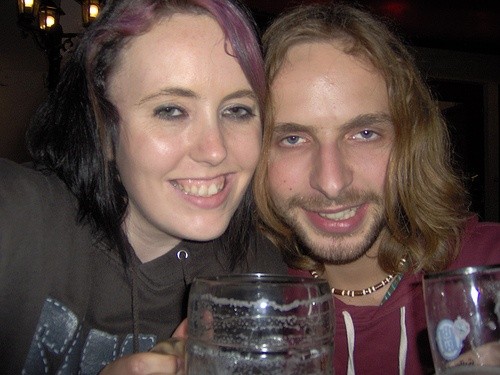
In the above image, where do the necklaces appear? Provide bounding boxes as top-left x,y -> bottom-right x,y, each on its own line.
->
306,254 -> 406,296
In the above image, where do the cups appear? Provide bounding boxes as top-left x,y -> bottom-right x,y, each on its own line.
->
183,273 -> 334,374
420,262 -> 500,375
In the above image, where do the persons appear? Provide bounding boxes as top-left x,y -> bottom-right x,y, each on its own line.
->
0,2 -> 287,375
252,7 -> 499,373
